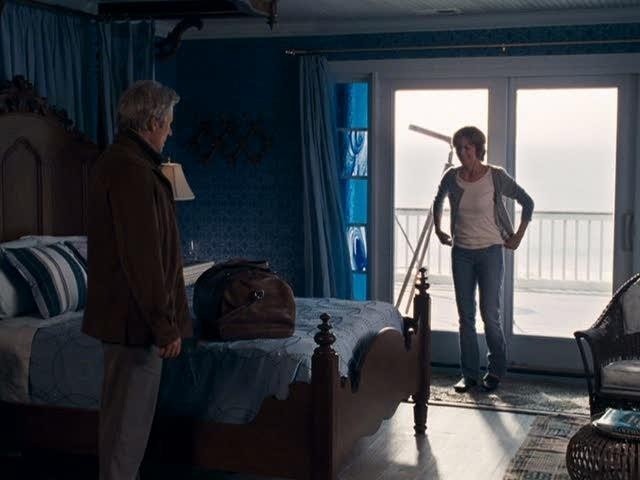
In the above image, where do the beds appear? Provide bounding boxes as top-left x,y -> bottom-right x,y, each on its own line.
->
1,72 -> 432,476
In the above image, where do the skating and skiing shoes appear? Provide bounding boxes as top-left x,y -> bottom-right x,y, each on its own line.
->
454,375 -> 476,391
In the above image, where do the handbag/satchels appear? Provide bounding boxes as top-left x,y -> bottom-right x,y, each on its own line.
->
193,262 -> 296,342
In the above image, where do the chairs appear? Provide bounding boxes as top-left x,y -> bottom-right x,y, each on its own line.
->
573,270 -> 640,421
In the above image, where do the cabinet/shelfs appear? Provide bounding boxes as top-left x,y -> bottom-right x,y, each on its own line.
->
322,68 -> 393,305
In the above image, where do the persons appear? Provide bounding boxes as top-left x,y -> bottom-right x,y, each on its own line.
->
433,126 -> 534,392
81,79 -> 187,480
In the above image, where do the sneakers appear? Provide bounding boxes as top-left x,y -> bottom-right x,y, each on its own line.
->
482,374 -> 499,390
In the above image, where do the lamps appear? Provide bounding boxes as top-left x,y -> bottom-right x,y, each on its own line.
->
158,154 -> 196,202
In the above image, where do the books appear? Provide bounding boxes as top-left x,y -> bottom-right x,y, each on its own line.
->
597,408 -> 640,435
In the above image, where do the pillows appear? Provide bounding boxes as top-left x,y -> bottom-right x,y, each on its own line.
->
0,233 -> 89,322
